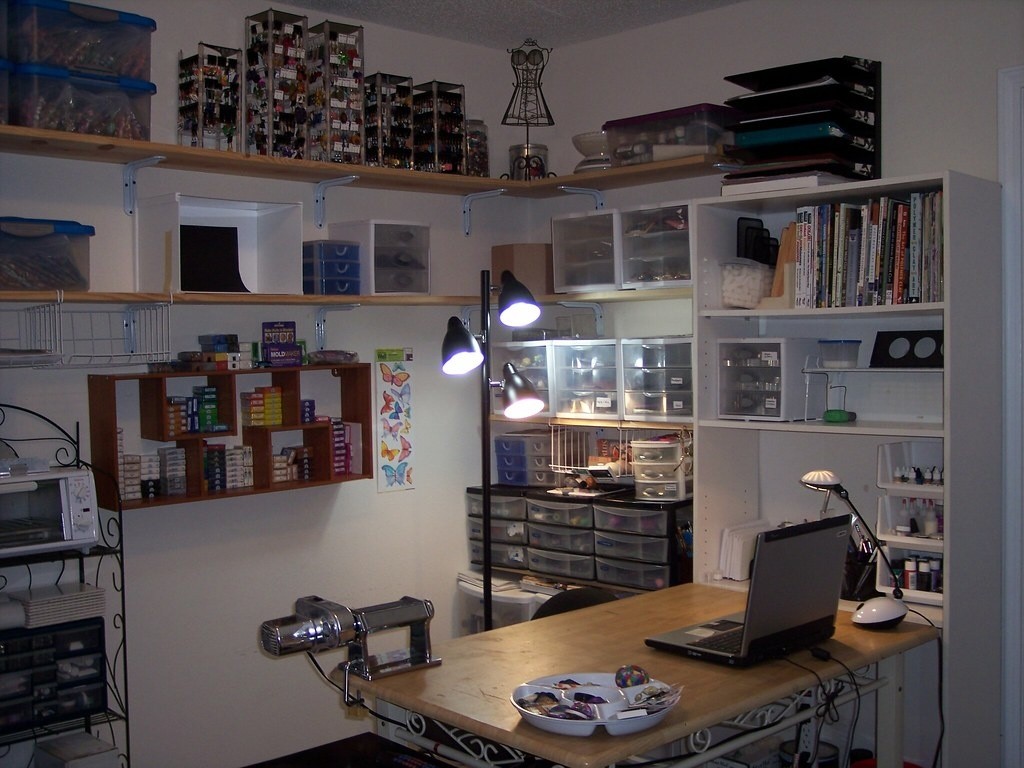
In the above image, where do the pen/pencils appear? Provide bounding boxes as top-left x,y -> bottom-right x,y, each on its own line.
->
849,525 -> 882,600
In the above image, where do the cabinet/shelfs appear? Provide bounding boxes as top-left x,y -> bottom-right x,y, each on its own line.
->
0,114 -> 722,300
0,405 -> 133,768
453,432 -> 686,592
720,52 -> 883,176
330,219 -> 432,294
538,200 -> 694,288
84,357 -> 379,510
483,336 -> 693,629
692,166 -> 1004,625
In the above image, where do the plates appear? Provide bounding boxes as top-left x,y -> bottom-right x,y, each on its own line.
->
509,671 -> 674,737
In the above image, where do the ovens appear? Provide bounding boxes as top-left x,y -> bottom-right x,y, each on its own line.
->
0,466 -> 100,558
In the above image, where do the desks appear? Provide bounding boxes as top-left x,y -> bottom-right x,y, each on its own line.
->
333,580 -> 942,768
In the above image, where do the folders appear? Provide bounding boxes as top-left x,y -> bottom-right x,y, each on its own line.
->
735,121 -> 848,148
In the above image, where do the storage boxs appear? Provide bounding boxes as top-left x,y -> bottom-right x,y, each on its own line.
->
602,103 -> 734,166
0,0 -> 157,144
717,336 -> 824,420
716,261 -> 774,308
0,216 -> 96,291
490,244 -> 552,293
302,238 -> 361,295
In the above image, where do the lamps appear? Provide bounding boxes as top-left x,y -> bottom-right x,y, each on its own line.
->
442,270 -> 542,633
800,469 -> 908,632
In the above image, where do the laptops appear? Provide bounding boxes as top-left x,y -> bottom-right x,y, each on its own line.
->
643,513 -> 853,668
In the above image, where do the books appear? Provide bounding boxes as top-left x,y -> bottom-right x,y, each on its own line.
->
908,188 -> 945,304
793,196 -> 910,307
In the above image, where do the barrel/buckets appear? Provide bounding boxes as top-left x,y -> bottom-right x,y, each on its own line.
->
508,143 -> 549,181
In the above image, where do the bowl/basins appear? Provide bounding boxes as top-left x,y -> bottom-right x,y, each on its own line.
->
817,339 -> 862,370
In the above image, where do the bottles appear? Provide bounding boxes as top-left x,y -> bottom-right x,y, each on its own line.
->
888,466 -> 944,591
